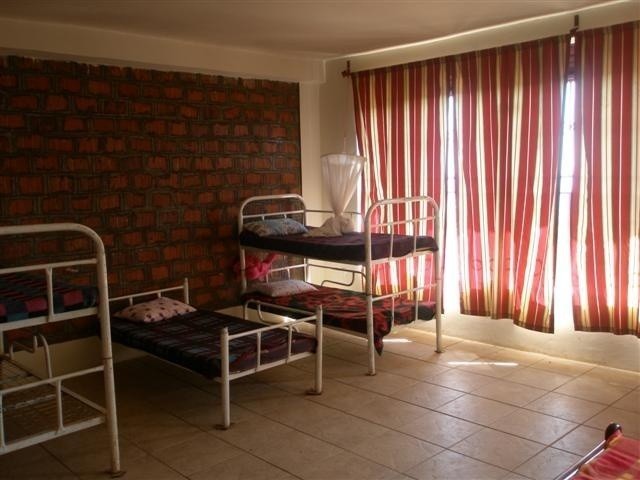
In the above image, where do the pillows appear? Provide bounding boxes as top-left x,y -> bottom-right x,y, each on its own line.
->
244,217 -> 308,238
113,296 -> 199,323
251,277 -> 318,298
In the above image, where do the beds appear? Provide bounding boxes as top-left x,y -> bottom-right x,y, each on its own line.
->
554,423 -> 640,480
236,194 -> 444,377
108,275 -> 324,430
0,220 -> 126,480
